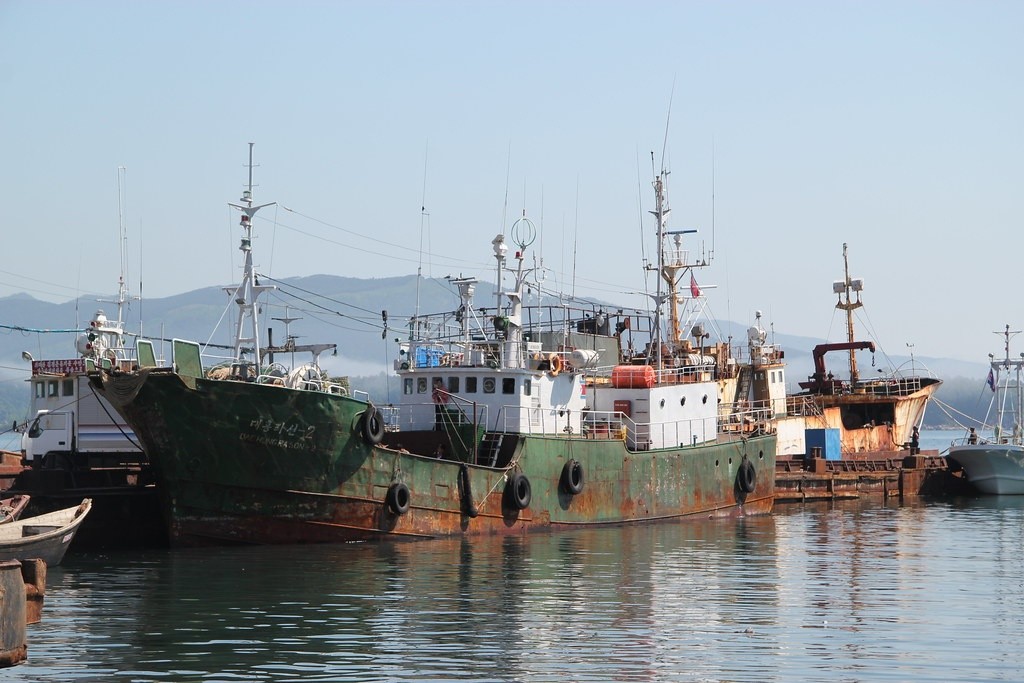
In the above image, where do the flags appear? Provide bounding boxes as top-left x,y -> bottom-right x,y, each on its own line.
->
985,368 -> 995,392
690,269 -> 700,298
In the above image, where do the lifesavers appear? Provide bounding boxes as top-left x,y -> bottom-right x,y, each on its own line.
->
534,353 -> 544,370
548,353 -> 560,376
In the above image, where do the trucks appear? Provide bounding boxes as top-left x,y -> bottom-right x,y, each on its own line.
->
12,375 -> 148,467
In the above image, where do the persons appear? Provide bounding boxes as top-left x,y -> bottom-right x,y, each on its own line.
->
909,426 -> 919,447
967,427 -> 977,444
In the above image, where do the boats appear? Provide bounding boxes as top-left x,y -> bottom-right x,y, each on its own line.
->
1,493 -> 31,523
945,320 -> 1024,496
717,237 -> 944,454
0,498 -> 94,565
85,141 -> 779,545
